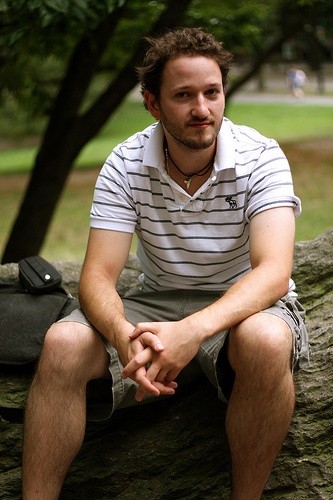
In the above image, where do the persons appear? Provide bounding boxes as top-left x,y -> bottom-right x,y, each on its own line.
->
286,62 -> 300,102
293,66 -> 308,100
18,26 -> 311,500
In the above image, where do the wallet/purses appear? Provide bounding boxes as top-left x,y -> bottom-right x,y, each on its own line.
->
16,251 -> 62,294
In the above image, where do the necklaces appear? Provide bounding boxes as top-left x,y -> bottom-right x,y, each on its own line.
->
165,147 -> 215,192
163,146 -> 170,176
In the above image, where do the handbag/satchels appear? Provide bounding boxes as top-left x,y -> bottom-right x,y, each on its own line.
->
0,282 -> 72,373
